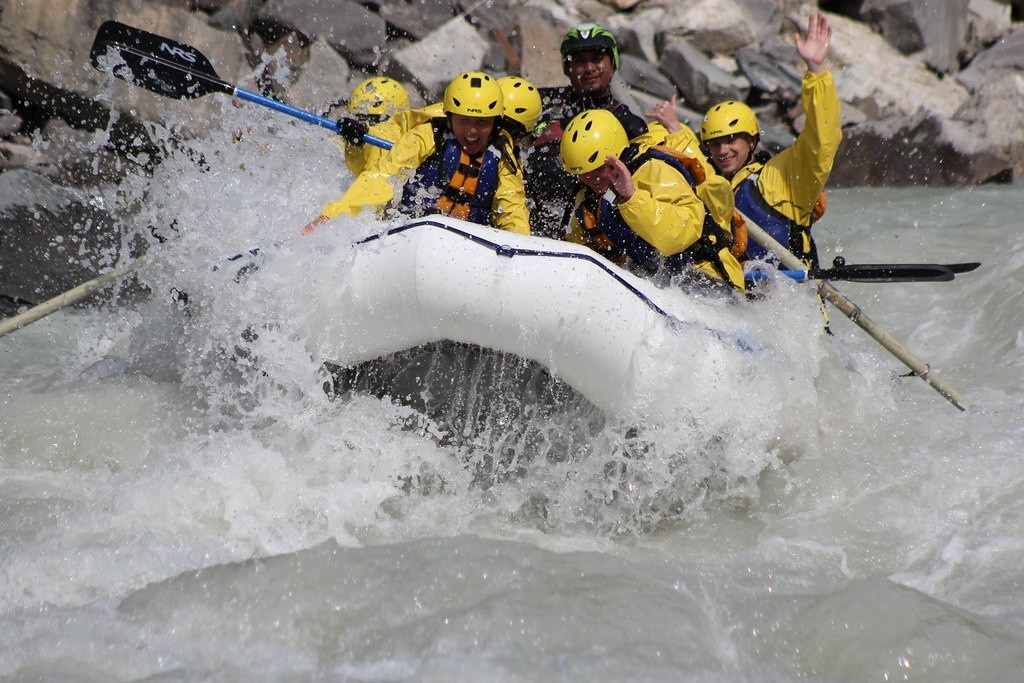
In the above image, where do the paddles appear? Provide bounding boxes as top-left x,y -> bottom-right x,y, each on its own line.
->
810,257 -> 982,283
89,21 -> 394,154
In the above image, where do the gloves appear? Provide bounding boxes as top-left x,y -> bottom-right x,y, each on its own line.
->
336,117 -> 369,147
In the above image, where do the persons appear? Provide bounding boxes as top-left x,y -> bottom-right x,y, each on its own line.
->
300,15 -> 842,336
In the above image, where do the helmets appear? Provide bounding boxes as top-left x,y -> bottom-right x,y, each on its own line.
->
645,121 -> 701,151
560,109 -> 630,177
347,76 -> 410,120
700,101 -> 761,153
495,75 -> 543,135
559,22 -> 619,75
442,71 -> 505,119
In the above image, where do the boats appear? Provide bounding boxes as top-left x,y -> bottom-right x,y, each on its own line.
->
199,214 -> 801,482
